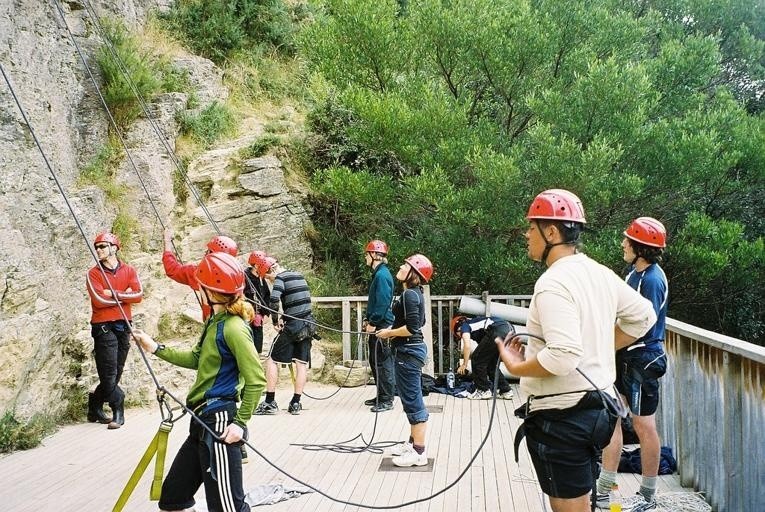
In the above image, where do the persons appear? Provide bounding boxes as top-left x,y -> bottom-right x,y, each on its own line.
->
362,239 -> 395,411
589,215 -> 671,511
492,188 -> 660,512
159,225 -> 250,465
238,250 -> 274,404
449,314 -> 515,401
375,254 -> 436,468
84,231 -> 144,430
125,250 -> 269,510
252,256 -> 316,416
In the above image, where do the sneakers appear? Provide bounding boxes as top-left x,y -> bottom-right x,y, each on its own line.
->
365,398 -> 376,405
493,389 -> 513,400
371,400 -> 394,412
288,402 -> 302,415
390,442 -> 413,455
391,448 -> 428,467
252,401 -> 278,415
623,491 -> 659,512
467,389 -> 492,400
589,490 -> 610,508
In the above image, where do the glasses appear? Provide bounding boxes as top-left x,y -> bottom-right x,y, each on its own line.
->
95,244 -> 111,250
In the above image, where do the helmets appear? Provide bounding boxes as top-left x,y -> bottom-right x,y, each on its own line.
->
194,252 -> 246,294
248,250 -> 267,264
526,188 -> 586,224
207,236 -> 238,257
256,256 -> 278,279
450,316 -> 466,337
365,239 -> 389,255
404,253 -> 434,283
94,233 -> 121,250
622,216 -> 667,248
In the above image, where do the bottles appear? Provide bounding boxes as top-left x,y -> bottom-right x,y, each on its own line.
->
609,483 -> 622,512
445,368 -> 455,390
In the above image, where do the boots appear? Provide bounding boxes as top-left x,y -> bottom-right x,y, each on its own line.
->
108,395 -> 124,429
87,393 -> 111,424
